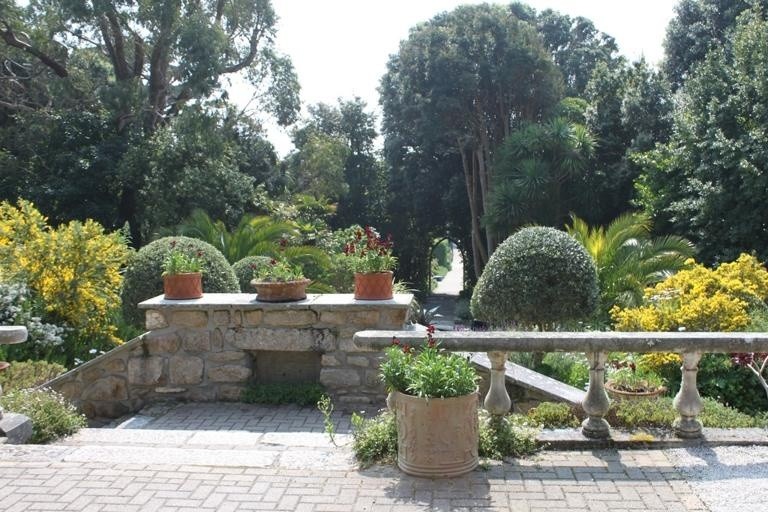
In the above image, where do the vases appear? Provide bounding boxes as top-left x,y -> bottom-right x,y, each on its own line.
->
250,276 -> 313,303
354,269 -> 396,301
161,269 -> 204,301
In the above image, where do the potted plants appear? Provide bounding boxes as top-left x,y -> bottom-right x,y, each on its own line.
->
374,345 -> 483,478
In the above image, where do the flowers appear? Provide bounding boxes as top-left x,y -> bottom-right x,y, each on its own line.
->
342,223 -> 400,276
159,239 -> 206,276
246,240 -> 303,281
